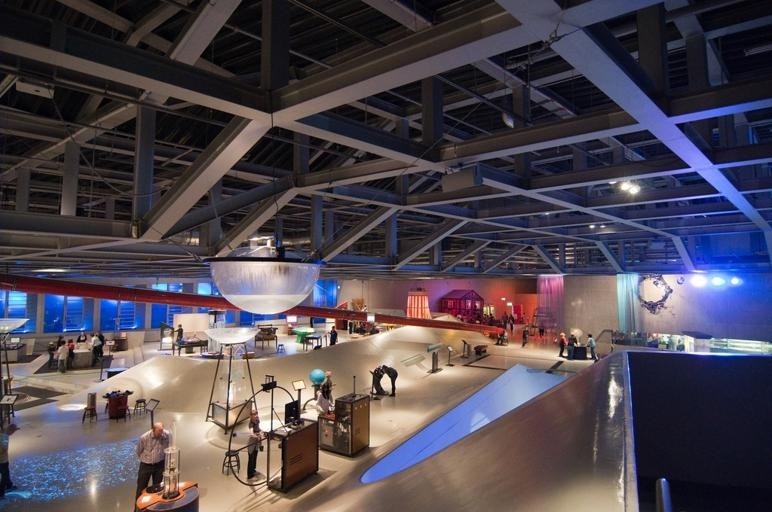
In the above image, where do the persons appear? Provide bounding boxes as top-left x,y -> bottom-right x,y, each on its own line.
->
458,309 -> 597,360
321,370 -> 337,403
249,407 -> 260,431
1,417 -> 21,499
246,426 -> 264,479
364,304 -> 368,312
328,326 -> 337,346
134,422 -> 170,512
349,319 -> 353,334
47,330 -> 106,373
316,384 -> 333,414
382,365 -> 397,396
174,324 -> 183,342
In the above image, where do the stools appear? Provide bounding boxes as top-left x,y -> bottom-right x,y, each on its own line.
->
81,405 -> 99,425
117,404 -> 133,422
133,397 -> 148,415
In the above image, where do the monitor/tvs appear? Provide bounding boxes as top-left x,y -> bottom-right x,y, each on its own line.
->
285,400 -> 300,425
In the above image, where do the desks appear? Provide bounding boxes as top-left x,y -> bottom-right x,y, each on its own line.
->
171,322 -> 329,358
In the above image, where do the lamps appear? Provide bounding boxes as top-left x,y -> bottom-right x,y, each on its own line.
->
202,18 -> 327,316
